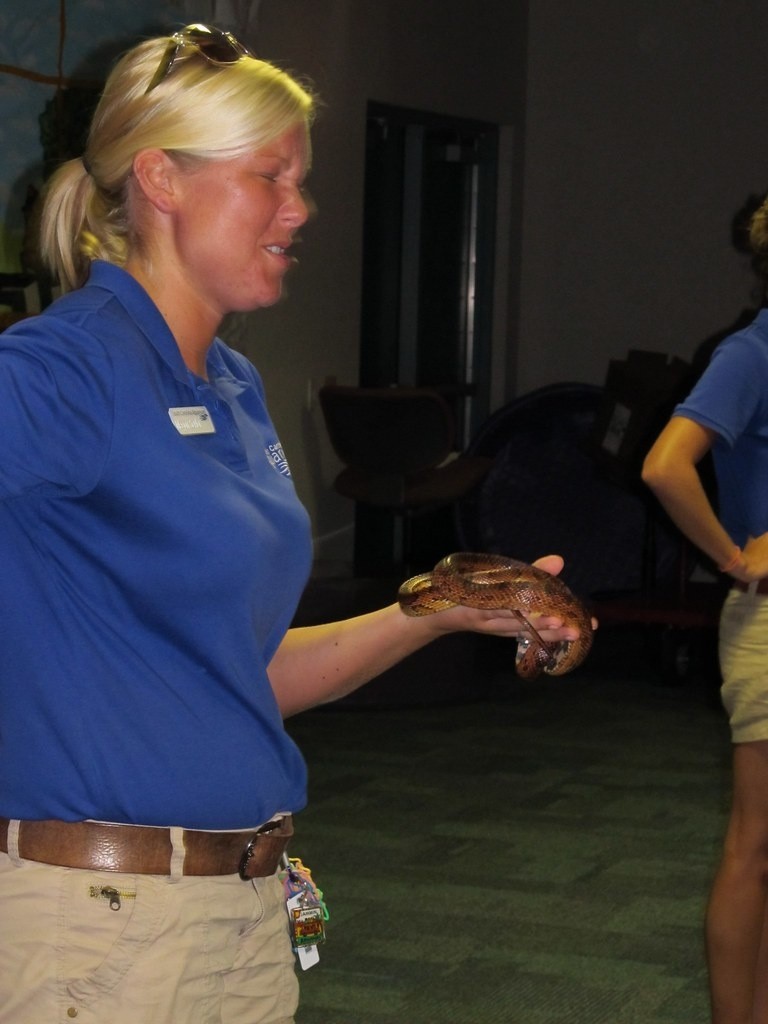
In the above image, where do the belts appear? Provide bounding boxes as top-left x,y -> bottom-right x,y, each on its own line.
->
732,577 -> 768,595
0,814 -> 300,880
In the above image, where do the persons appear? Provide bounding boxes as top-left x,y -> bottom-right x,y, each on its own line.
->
2,24 -> 609,1022
641,192 -> 767,1024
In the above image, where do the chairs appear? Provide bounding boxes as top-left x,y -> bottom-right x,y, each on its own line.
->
450,381 -> 721,687
322,376 -> 491,616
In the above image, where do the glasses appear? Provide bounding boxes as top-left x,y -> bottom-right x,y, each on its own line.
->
147,24 -> 254,96
